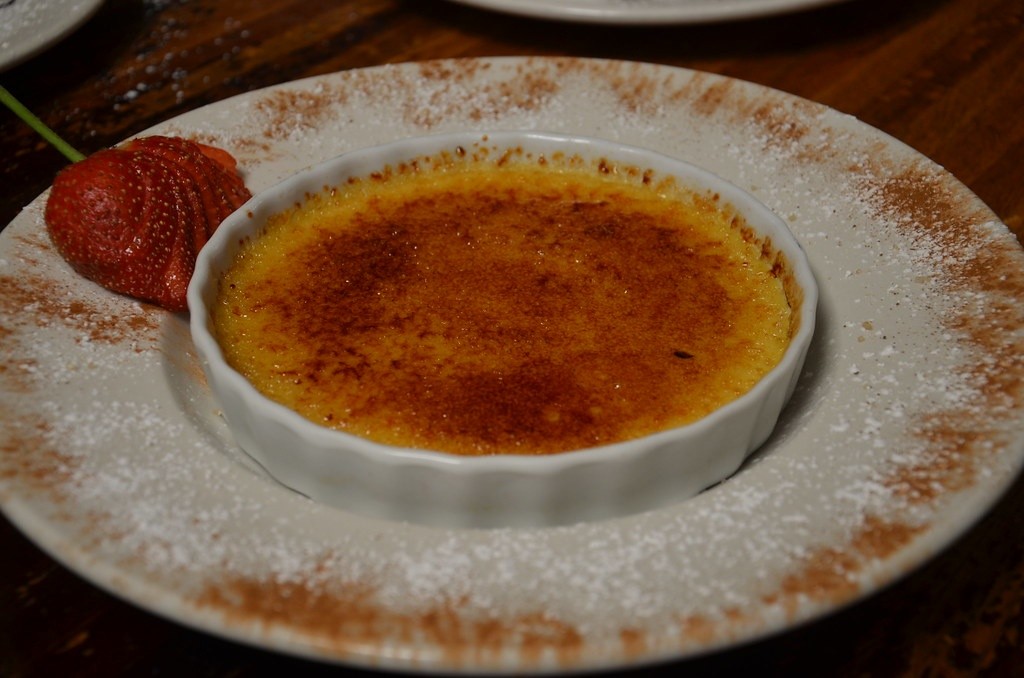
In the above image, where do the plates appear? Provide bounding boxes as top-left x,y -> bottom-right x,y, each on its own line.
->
461,1 -> 832,24
0,0 -> 102,64
0,55 -> 1024,677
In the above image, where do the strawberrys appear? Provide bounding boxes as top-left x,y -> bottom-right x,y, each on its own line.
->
44,135 -> 261,314
